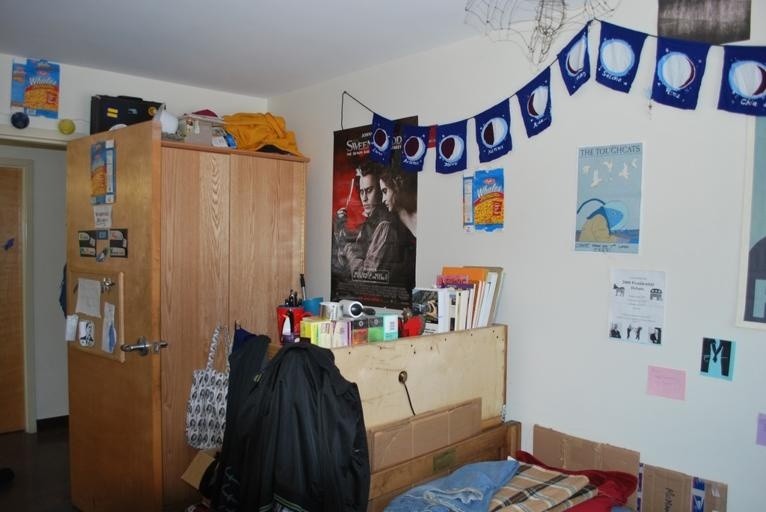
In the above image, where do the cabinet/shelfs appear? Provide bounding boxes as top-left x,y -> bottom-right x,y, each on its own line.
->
155,141 -> 308,501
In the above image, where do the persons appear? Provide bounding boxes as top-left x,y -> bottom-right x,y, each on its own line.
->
331,163 -> 415,286
378,165 -> 417,238
80,322 -> 94,341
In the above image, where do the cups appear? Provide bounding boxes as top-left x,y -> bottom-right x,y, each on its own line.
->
276,305 -> 312,345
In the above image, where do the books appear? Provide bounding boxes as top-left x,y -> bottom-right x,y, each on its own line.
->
412,266 -> 505,335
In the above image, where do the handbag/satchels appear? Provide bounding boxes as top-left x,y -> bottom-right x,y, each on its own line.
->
184,325 -> 231,450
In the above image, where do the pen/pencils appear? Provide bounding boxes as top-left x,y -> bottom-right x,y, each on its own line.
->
300,274 -> 307,299
285,290 -> 297,306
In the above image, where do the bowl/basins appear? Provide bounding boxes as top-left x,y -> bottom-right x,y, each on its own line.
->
150,103 -> 178,134
